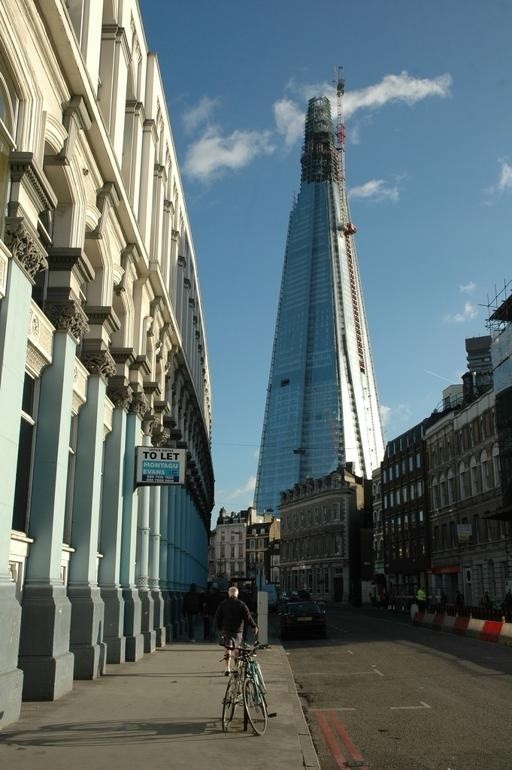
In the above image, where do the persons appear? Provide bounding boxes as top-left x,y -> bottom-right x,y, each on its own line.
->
505,589 -> 512,609
217,586 -> 259,675
414,583 -> 426,612
286,582 -> 310,602
182,582 -> 218,643
481,591 -> 490,607
455,590 -> 464,608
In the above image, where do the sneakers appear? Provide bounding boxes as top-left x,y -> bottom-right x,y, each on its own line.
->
225,667 -> 231,676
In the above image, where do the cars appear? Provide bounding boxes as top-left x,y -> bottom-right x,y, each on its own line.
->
280,601 -> 328,639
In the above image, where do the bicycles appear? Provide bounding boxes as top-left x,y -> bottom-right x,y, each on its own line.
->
219,641 -> 277,736
